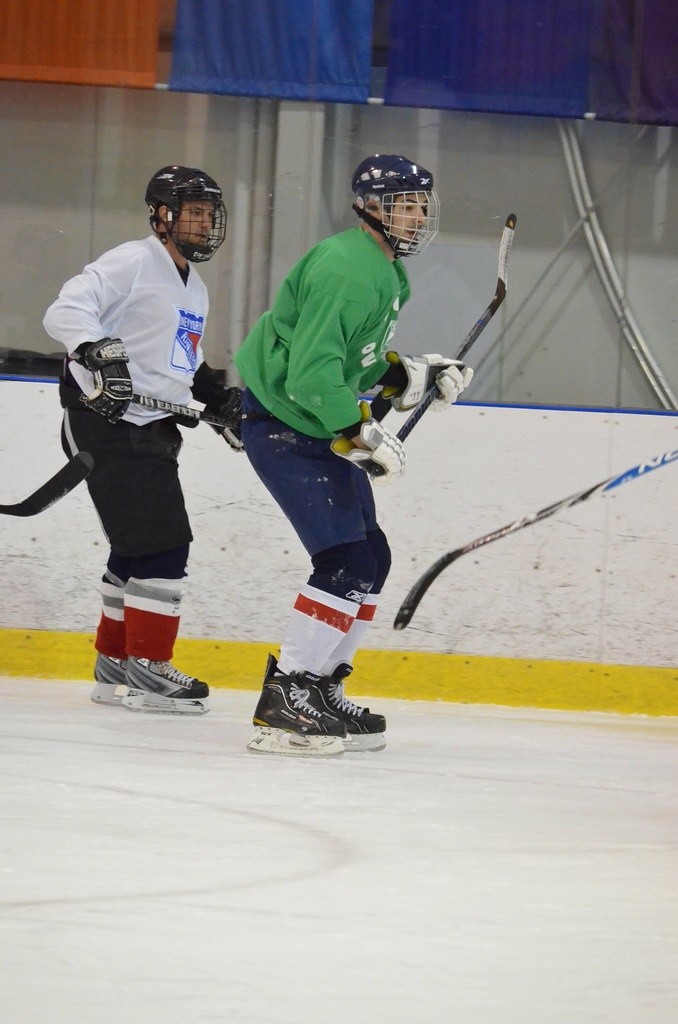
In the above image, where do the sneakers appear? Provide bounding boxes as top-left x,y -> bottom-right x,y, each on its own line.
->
121,655 -> 211,716
247,653 -> 347,759
307,661 -> 387,753
90,651 -> 128,707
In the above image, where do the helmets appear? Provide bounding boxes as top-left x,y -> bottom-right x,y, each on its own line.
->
351,154 -> 439,257
144,165 -> 227,263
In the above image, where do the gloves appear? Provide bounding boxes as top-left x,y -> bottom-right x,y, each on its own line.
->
330,400 -> 406,482
79,337 -> 134,425
380,350 -> 474,412
204,387 -> 245,452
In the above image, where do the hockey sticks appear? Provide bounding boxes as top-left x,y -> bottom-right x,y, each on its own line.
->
370,211 -> 521,480
0,450 -> 96,519
131,391 -> 236,431
390,446 -> 678,630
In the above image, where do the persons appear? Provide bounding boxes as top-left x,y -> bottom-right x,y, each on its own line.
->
41,164 -> 247,717
236,153 -> 475,758
